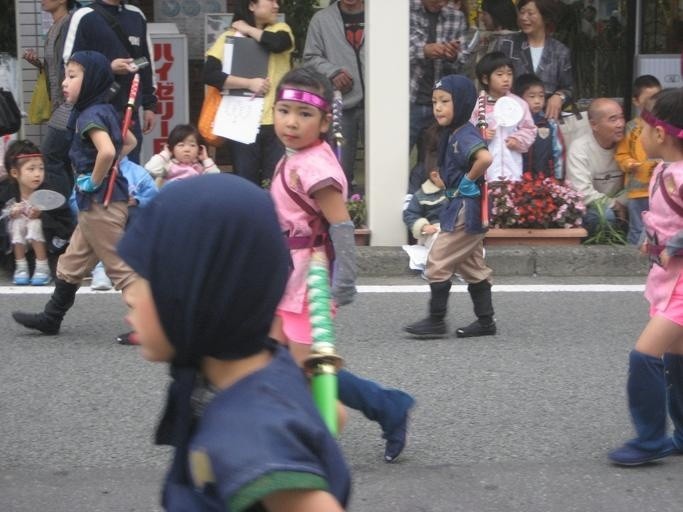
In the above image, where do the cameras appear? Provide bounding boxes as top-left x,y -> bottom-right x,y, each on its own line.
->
127,55 -> 151,70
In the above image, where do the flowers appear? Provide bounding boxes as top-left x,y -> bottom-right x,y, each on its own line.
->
486,171 -> 587,229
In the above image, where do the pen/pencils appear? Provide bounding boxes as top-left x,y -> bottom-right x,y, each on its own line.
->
421,231 -> 428,237
249,77 -> 271,103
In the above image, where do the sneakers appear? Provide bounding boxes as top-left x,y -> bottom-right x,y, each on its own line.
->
456,320 -> 497,337
30,272 -> 53,286
14,269 -> 29,285
405,318 -> 445,334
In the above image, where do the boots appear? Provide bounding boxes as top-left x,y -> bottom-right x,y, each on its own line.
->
339,369 -> 414,461
610,350 -> 683,465
14,279 -> 76,335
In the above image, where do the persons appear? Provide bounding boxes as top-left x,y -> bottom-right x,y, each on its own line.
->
603,80 -> 683,467
400,69 -> 503,340
410,1 -> 683,280
2,2 -> 363,287
109,167 -> 356,510
10,44 -> 145,348
256,62 -> 417,464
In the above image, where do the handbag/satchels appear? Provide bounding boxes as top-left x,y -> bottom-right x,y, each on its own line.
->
28,67 -> 50,124
197,85 -> 227,145
0,88 -> 20,135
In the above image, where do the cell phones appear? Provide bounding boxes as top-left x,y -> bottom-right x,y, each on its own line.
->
444,38 -> 462,46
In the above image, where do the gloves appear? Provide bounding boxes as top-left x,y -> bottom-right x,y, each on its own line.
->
77,172 -> 97,195
327,219 -> 357,306
455,176 -> 481,197
